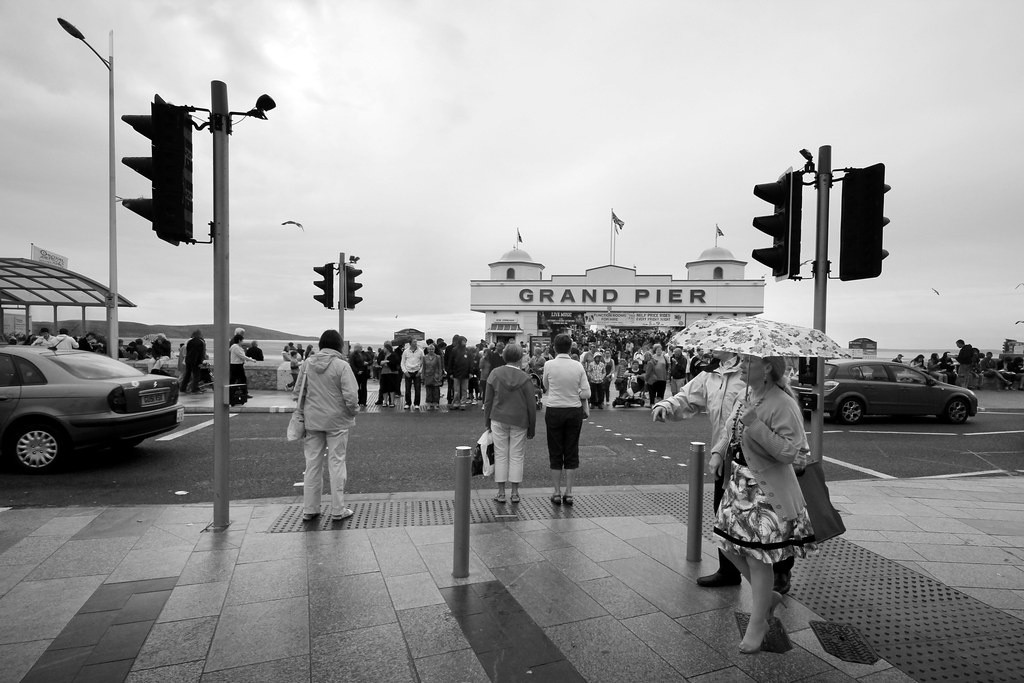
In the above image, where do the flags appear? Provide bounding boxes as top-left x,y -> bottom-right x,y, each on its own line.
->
717,227 -> 723,237
612,213 -> 625,235
517,230 -> 522,245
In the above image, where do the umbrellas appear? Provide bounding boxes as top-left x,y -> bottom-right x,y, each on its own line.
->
665,316 -> 854,400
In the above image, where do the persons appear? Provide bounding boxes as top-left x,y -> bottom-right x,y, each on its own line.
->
287,330 -> 358,525
892,339 -> 1024,395
280,326 -> 721,408
0,323 -> 264,400
542,336 -> 591,507
709,354 -> 820,651
650,347 -> 811,586
484,344 -> 537,503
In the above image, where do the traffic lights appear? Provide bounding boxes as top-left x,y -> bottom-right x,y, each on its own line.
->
839,163 -> 891,281
752,167 -> 793,282
121,93 -> 193,246
313,263 -> 333,309
223,384 -> 248,407
344,263 -> 363,310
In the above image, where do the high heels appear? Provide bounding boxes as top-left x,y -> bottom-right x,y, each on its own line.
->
770,590 -> 787,616
739,621 -> 771,653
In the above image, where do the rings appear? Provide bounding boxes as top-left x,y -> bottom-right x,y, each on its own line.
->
740,412 -> 743,416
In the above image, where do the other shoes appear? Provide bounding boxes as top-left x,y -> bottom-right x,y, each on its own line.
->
389,404 -> 396,407
404,405 -> 410,409
332,510 -> 353,520
696,572 -> 741,587
774,572 -> 792,594
302,514 -> 316,520
382,404 -> 388,407
466,400 -> 478,405
415,405 -> 419,409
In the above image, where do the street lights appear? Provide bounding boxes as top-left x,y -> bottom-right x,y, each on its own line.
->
59,17 -> 118,365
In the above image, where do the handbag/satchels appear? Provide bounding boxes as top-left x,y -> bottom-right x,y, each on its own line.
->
246,346 -> 263,361
471,445 -> 483,477
477,429 -> 495,476
287,409 -> 305,441
795,459 -> 847,543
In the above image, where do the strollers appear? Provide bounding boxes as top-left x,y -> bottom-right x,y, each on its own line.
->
612,376 -> 647,408
186,355 -> 215,395
530,373 -> 543,410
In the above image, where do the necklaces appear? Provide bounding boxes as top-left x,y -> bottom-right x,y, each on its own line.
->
729,391 -> 765,444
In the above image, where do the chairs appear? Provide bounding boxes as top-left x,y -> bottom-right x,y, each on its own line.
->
873,370 -> 886,381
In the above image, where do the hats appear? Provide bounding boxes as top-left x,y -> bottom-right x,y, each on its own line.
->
594,352 -> 602,359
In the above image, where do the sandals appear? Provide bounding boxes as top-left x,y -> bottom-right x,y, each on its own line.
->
552,495 -> 561,504
563,495 -> 573,505
494,494 -> 507,502
511,494 -> 520,502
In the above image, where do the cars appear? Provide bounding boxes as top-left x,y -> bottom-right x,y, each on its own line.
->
790,358 -> 978,426
0,343 -> 185,477
